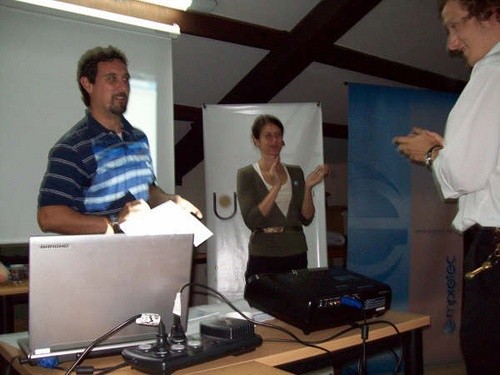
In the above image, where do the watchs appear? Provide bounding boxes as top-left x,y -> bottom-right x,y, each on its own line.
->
107,213 -> 121,234
423,144 -> 442,168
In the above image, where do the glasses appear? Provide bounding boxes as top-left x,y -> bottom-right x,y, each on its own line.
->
447,13 -> 474,32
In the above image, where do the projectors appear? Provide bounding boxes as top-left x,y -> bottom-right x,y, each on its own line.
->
244,267 -> 393,334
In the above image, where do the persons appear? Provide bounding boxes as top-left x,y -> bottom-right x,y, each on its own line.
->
391,0 -> 500,375
236,115 -> 329,294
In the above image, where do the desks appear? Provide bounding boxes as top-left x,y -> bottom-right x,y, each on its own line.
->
0,290 -> 431,375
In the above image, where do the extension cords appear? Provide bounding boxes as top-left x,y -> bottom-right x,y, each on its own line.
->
122,316 -> 263,375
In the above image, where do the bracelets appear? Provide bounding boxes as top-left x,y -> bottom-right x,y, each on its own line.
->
36,46 -> 205,234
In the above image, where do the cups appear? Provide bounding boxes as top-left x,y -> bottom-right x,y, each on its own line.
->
9,264 -> 25,285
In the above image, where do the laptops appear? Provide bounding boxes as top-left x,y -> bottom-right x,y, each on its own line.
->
18,232 -> 194,366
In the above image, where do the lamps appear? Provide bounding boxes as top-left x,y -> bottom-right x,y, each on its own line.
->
14,0 -> 192,41
